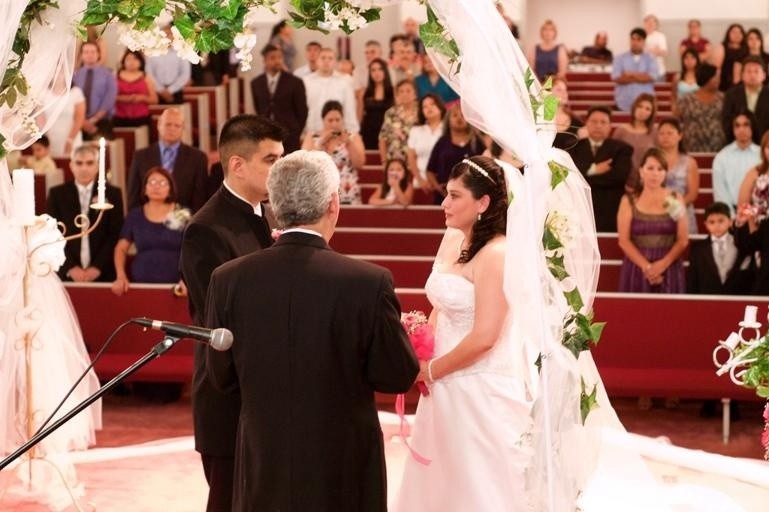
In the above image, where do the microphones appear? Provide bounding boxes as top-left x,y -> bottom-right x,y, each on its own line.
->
129,315 -> 236,353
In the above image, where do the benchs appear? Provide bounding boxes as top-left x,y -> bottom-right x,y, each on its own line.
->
33,71 -> 769,446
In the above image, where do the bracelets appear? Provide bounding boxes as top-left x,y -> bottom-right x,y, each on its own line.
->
426,358 -> 435,382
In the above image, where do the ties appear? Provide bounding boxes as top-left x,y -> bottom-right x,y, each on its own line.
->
716,238 -> 725,269
84,68 -> 92,115
80,188 -> 91,269
162,148 -> 173,174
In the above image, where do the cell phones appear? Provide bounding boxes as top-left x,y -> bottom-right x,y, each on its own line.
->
741,207 -> 756,219
329,130 -> 342,139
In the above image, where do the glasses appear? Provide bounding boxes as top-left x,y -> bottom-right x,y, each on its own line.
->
150,178 -> 169,187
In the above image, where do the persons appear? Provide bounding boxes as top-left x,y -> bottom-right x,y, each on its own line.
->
24,12 -> 208,210
203,150 -> 415,510
685,201 -> 750,420
174,113 -> 293,512
528,13 -> 769,234
39,142 -> 121,286
110,164 -> 191,298
397,154 -> 583,512
613,148 -> 692,413
249,20 -> 491,208
